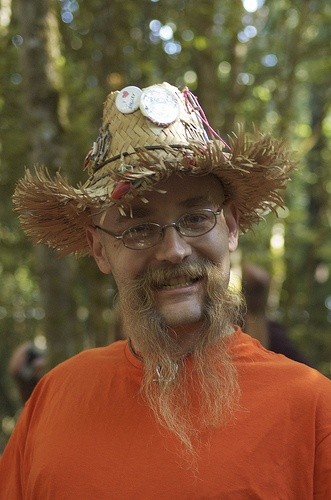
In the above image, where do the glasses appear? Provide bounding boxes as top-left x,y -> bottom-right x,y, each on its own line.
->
94,197 -> 230,250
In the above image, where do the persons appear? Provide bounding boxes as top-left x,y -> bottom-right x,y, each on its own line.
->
1,80 -> 331,500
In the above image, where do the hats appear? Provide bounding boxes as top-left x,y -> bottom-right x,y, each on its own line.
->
13,82 -> 295,257
